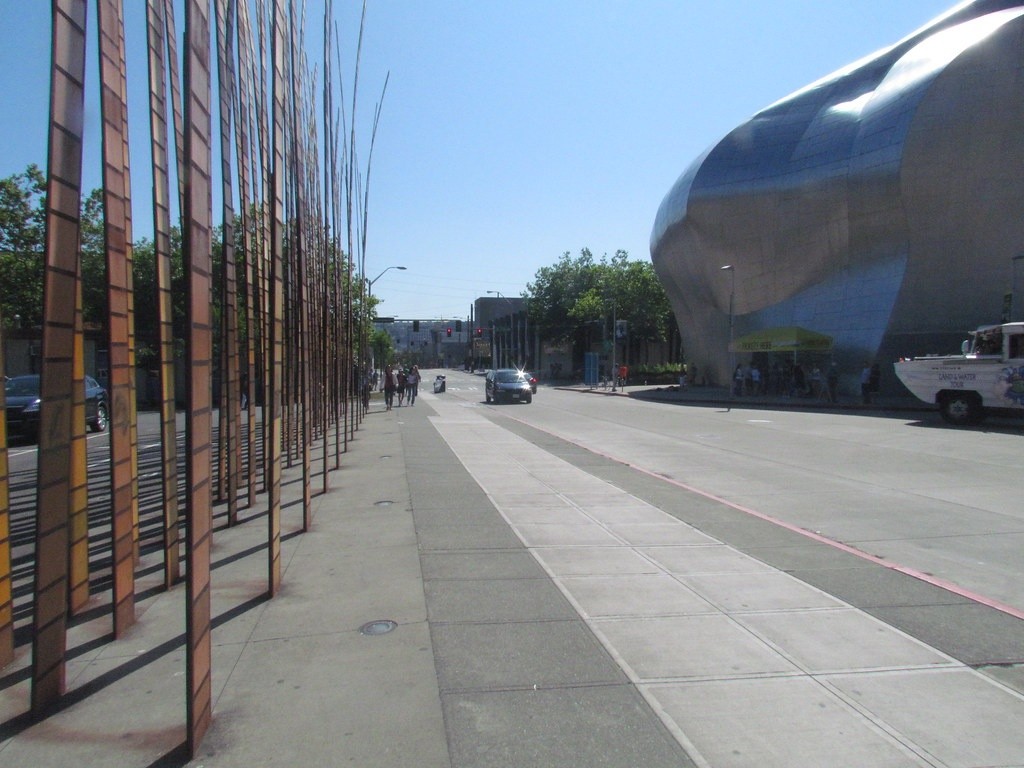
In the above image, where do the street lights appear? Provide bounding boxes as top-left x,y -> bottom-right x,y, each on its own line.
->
487,290 -> 515,367
604,298 -> 617,391
721,264 -> 735,400
367,265 -> 407,346
1011,254 -> 1023,322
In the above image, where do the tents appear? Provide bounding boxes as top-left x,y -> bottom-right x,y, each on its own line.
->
727,326 -> 833,365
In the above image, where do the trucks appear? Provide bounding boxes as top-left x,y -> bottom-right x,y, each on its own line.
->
893,322 -> 1024,426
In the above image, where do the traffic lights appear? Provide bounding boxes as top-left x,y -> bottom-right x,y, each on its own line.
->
477,328 -> 483,335
447,328 -> 452,337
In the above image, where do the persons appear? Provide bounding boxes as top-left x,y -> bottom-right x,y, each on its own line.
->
369,369 -> 378,391
380,364 -> 421,410
783,360 -> 821,398
735,364 -> 743,394
612,363 -> 619,391
679,369 -> 687,389
861,361 -> 878,402
748,359 -> 769,395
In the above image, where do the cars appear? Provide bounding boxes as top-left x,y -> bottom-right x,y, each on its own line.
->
4,374 -> 110,442
486,369 -> 537,404
